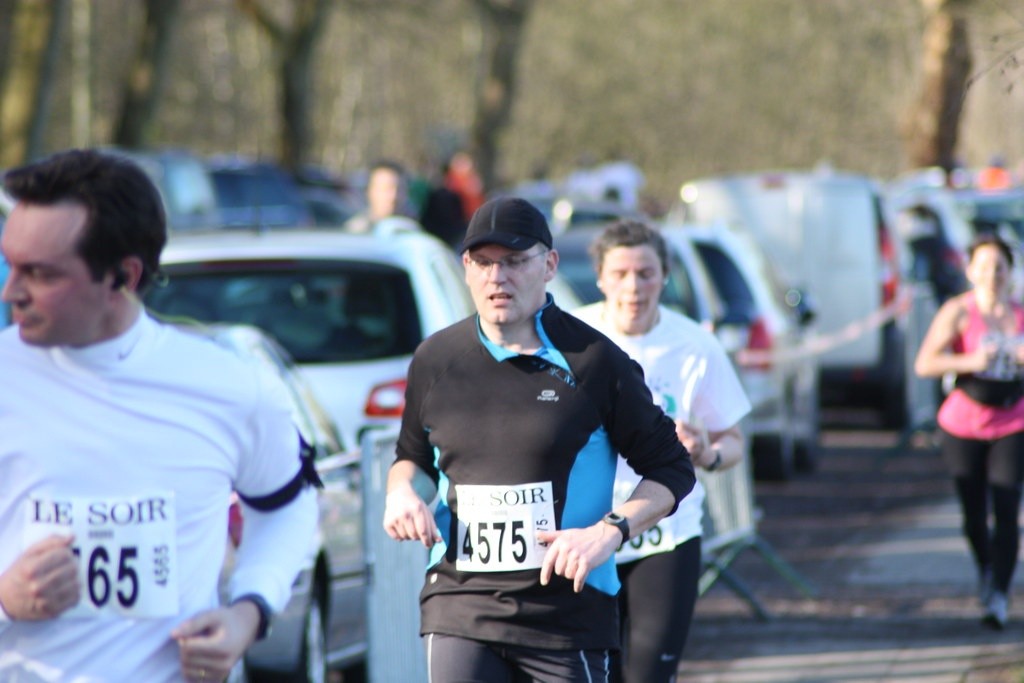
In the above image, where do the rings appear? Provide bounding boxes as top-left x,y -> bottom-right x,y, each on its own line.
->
199,669 -> 205,683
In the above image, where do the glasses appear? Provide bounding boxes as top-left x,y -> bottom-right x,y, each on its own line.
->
470,250 -> 546,273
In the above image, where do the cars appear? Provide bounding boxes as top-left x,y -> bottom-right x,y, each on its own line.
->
76,148 -> 1024,479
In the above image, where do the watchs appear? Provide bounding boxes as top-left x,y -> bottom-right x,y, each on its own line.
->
601,512 -> 629,544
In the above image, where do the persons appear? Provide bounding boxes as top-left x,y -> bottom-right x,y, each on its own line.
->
564,220 -> 755,683
345,161 -> 412,235
0,148 -> 319,683
915,236 -> 1024,628
421,149 -> 492,248
383,196 -> 697,683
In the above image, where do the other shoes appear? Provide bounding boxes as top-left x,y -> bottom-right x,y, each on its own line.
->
978,568 -> 992,601
982,595 -> 1009,629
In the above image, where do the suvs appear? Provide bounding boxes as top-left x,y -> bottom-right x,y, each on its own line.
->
134,219 -> 485,680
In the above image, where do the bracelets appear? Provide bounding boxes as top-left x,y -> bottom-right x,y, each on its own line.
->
706,453 -> 721,471
240,595 -> 271,640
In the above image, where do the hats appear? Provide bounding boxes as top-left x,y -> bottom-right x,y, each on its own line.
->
459,198 -> 553,254
